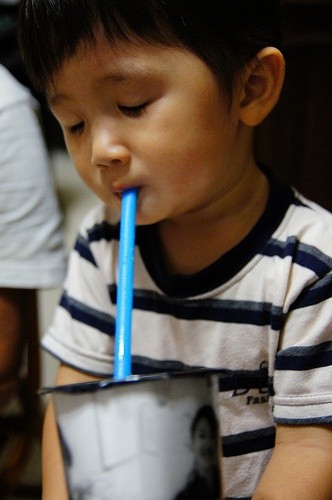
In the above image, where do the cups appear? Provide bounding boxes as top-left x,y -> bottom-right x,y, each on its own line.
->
41,364 -> 231,500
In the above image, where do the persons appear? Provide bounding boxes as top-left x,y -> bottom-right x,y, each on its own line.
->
14,0 -> 332,500
0,63 -> 66,385
